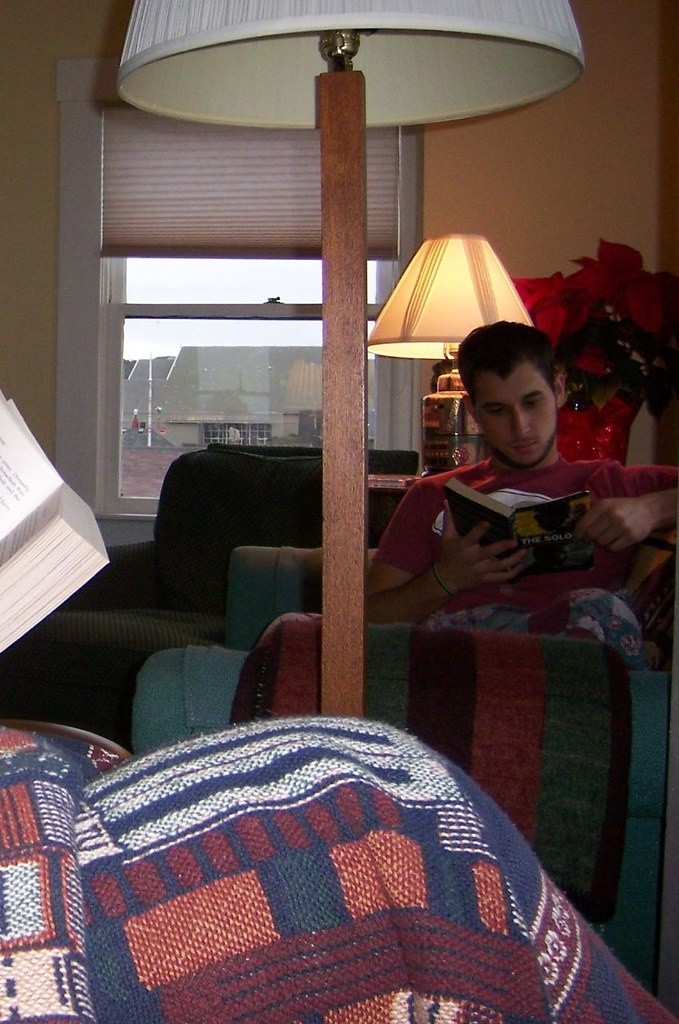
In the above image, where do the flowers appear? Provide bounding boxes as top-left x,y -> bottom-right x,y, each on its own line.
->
517,239 -> 679,424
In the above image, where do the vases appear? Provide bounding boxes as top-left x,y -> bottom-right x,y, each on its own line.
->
553,390 -> 646,460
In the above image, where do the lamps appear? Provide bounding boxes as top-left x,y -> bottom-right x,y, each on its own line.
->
112,1 -> 585,716
368,231 -> 533,476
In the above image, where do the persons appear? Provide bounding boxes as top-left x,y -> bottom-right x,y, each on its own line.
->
364,322 -> 678,672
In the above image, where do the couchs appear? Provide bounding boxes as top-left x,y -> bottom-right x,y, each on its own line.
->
0,447 -> 679,1022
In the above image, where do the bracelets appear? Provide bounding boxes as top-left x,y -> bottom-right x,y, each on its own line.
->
432,565 -> 458,596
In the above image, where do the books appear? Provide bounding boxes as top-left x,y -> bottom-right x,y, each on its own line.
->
444,476 -> 598,584
